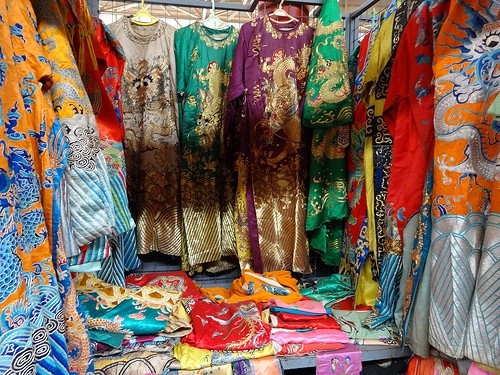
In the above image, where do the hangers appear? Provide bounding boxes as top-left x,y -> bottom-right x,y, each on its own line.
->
260,0 -> 299,24
203,0 -> 229,29
130,0 -> 158,26
